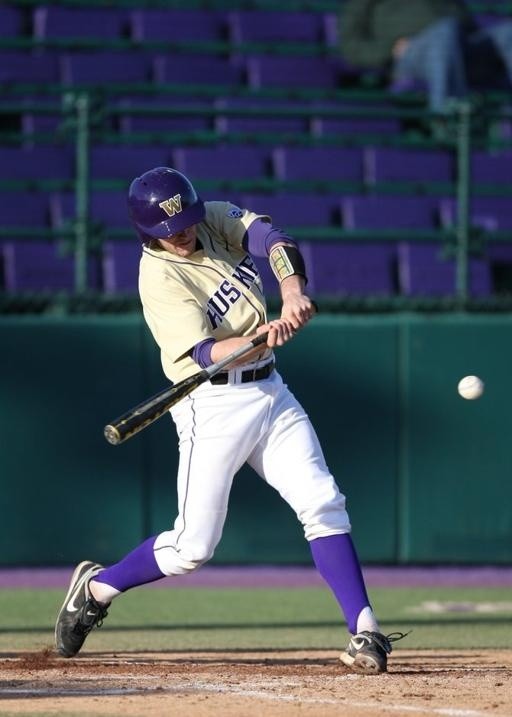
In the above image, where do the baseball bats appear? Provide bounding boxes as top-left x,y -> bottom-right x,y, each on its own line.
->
104,299 -> 320,446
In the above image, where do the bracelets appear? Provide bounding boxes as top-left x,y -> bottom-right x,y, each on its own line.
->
268,246 -> 308,286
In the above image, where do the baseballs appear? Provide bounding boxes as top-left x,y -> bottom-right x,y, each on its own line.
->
458,374 -> 485,401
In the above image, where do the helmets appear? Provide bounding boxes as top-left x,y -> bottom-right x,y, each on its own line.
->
126,165 -> 206,245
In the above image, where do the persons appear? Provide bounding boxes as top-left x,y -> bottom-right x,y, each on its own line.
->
55,166 -> 412,674
337,1 -> 510,134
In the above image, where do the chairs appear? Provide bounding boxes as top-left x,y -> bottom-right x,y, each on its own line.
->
0,0 -> 512,295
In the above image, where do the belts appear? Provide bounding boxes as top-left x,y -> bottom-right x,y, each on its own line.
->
203,356 -> 275,387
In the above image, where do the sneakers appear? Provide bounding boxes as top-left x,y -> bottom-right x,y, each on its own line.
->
339,630 -> 392,676
54,560 -> 112,658
